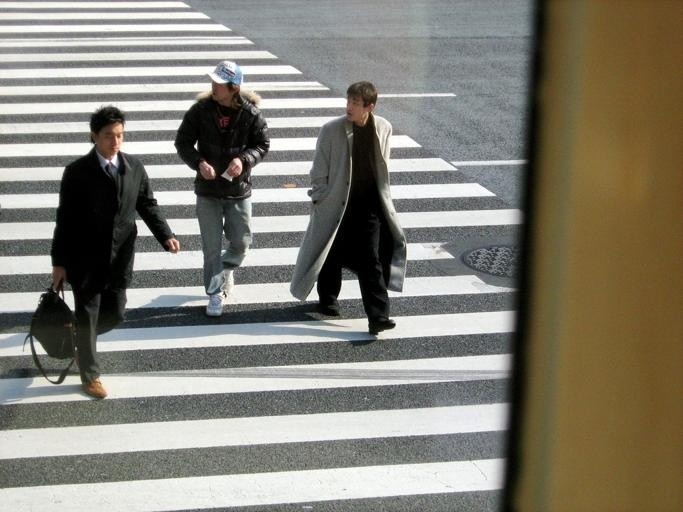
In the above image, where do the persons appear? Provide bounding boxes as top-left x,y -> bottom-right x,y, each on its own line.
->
173,58 -> 271,320
288,81 -> 408,334
50,104 -> 181,400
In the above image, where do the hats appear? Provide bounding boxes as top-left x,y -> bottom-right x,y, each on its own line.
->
208,61 -> 242,85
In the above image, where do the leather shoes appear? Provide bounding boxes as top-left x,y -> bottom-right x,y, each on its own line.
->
83,377 -> 108,398
320,299 -> 340,316
369,318 -> 397,334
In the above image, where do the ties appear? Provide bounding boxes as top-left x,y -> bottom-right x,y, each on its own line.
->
105,163 -> 114,179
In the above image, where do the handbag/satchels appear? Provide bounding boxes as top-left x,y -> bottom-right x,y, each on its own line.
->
30,289 -> 75,359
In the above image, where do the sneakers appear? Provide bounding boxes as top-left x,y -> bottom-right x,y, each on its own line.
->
206,269 -> 234,317
